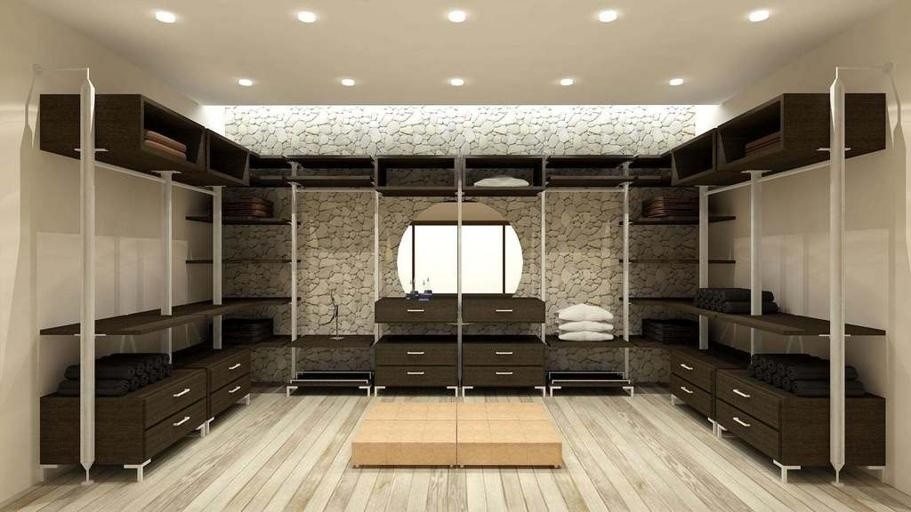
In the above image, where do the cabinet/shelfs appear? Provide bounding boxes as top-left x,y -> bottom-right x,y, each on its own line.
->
632,296 -> 887,483
372,296 -> 459,398
715,92 -> 886,187
460,154 -> 544,186
40,94 -> 206,186
255,154 -> 371,185
547,156 -> 669,186
373,156 -> 457,186
461,295 -> 546,397
352,398 -> 562,469
40,296 -> 303,486
179,127 -> 249,187
672,130 -> 725,188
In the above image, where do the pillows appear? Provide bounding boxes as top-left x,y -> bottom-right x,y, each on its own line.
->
558,303 -> 614,322
560,321 -> 614,332
556,331 -> 614,342
